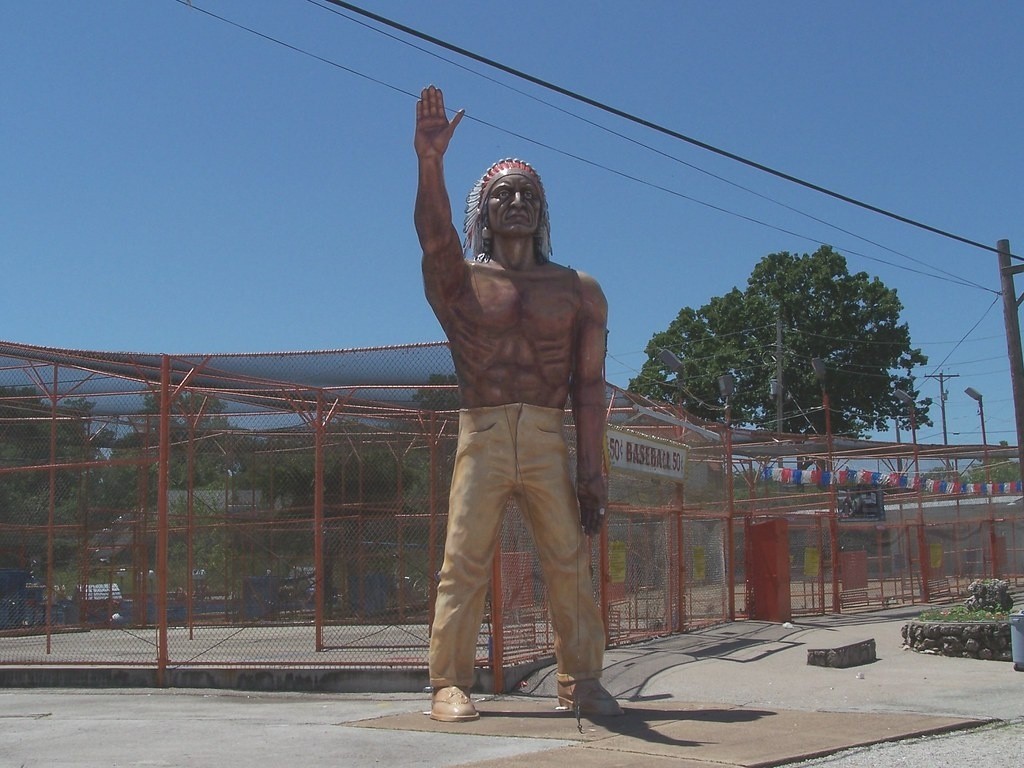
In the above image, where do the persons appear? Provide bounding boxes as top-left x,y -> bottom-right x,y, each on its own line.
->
413,84 -> 627,724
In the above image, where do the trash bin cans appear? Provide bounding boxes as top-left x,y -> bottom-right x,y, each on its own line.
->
1009,609 -> 1024,672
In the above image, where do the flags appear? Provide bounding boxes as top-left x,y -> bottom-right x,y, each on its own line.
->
761,466 -> 1022,496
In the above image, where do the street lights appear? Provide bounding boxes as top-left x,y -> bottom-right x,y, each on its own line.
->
810,355 -> 841,615
893,388 -> 931,603
716,375 -> 736,622
963,386 -> 999,579
657,349 -> 687,632
